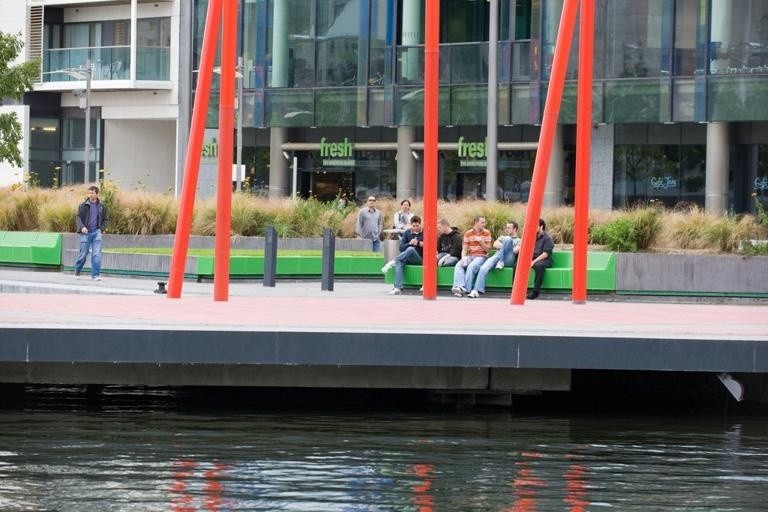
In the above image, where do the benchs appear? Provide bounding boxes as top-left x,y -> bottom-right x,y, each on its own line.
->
383,240 -> 617,295
0,231 -> 63,270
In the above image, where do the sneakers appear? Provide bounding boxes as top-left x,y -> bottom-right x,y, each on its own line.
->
496,261 -> 504,269
381,264 -> 392,273
460,285 -> 470,293
387,288 -> 403,295
452,286 -> 463,297
527,290 -> 539,299
92,276 -> 101,281
468,290 -> 480,298
74,271 -> 80,279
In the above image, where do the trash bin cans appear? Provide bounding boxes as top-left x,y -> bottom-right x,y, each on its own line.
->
383,229 -> 405,266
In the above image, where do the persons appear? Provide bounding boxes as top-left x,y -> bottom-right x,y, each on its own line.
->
338,192 -> 352,210
74,186 -> 108,281
356,196 -> 384,251
381,200 -> 554,299
